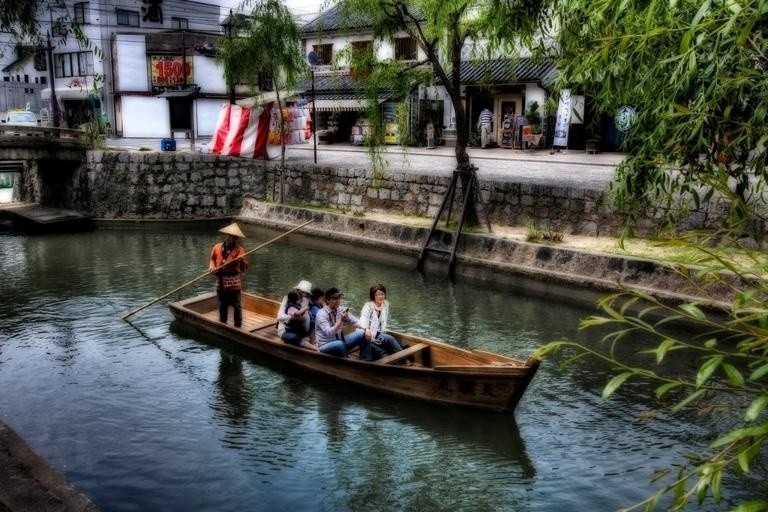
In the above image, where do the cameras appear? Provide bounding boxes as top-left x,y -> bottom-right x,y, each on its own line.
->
378,337 -> 385,341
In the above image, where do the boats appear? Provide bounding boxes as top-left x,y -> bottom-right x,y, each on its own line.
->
167,287 -> 541,415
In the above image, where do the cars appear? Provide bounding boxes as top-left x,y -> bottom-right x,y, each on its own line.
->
2,111 -> 40,136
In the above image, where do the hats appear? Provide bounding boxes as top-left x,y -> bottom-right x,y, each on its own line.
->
324,287 -> 345,299
293,280 -> 313,296
218,222 -> 247,242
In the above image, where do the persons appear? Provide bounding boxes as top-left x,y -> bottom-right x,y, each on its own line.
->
276,280 -> 318,351
315,286 -> 372,362
309,288 -> 324,331
357,284 -> 410,366
285,291 -> 310,343
207,233 -> 250,328
476,107 -> 494,148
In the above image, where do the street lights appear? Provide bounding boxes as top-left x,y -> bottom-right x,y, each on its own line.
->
219,9 -> 240,104
